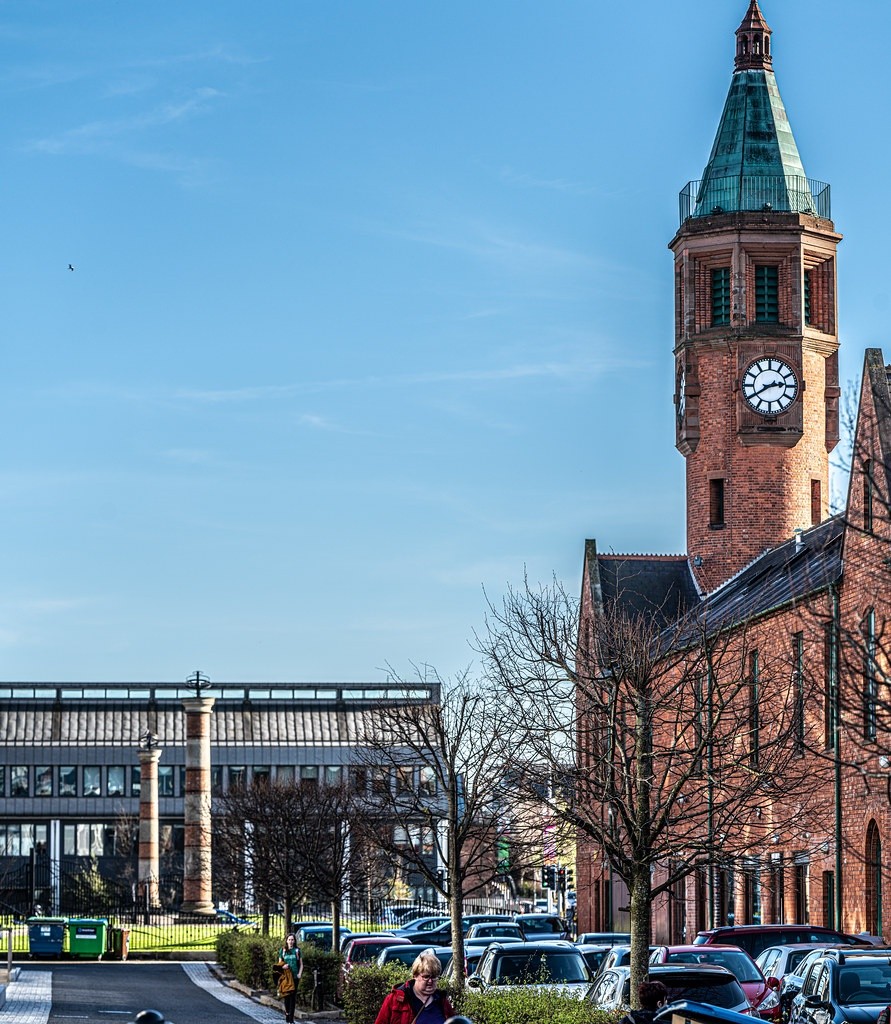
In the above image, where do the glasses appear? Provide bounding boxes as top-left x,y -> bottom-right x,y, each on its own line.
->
418,973 -> 442,982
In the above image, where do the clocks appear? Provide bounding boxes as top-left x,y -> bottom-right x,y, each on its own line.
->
742,358 -> 799,416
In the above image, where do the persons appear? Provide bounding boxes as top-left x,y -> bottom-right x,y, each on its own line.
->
374,955 -> 454,1024
617,980 -> 670,1024
272,933 -> 304,1024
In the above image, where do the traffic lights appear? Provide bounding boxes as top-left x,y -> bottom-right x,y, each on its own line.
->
557,866 -> 567,891
541,865 -> 550,887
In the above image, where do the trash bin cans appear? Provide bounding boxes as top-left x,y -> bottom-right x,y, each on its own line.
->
27,917 -> 64,960
69,919 -> 108,962
114,929 -> 128,961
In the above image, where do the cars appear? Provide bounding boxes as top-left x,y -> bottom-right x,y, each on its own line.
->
286,904 -> 891,1024
214,909 -> 253,925
